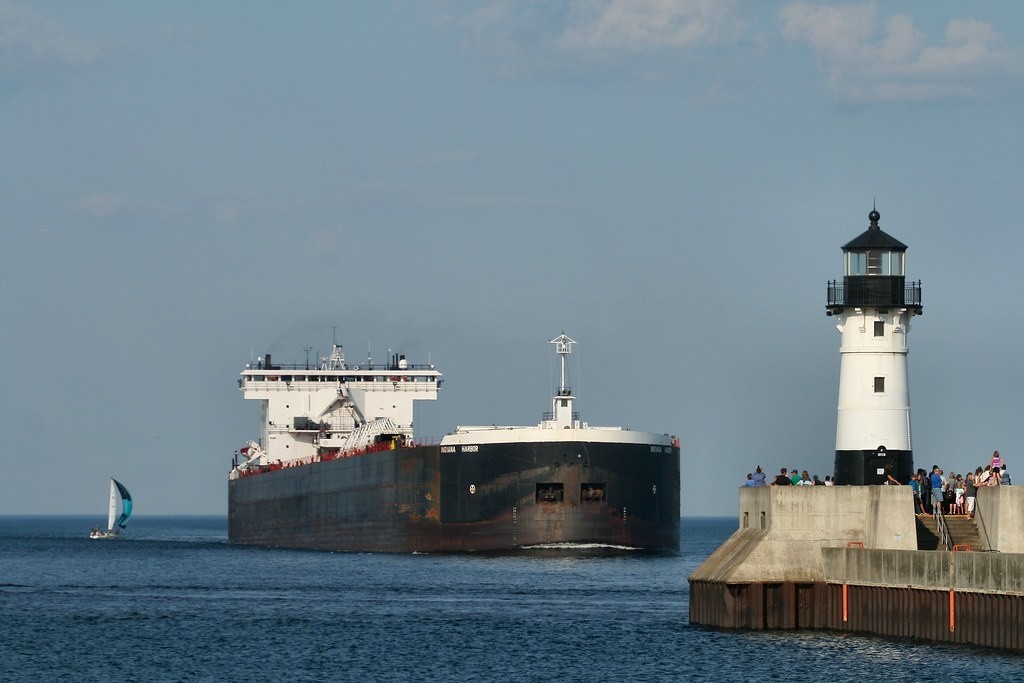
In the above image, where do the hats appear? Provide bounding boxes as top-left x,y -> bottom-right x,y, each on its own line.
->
955,474 -> 961,479
790,470 -> 798,473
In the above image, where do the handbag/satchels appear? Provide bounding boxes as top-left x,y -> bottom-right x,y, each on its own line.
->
946,491 -> 954,499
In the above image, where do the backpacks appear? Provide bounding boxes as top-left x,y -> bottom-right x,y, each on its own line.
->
1001,470 -> 1009,485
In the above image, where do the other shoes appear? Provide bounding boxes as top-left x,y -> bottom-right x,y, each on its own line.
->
922,513 -> 929,515
947,512 -> 952,515
968,517 -> 973,519
953,512 -> 955,515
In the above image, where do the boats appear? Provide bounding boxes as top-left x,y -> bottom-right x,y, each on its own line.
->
228,332 -> 681,559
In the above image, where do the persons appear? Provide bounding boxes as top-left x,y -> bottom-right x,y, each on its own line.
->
770,467 -> 834,487
908,450 -> 1012,520
883,463 -> 901,485
744,465 -> 767,487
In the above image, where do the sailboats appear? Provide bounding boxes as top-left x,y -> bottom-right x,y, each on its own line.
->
90,478 -> 133,540
